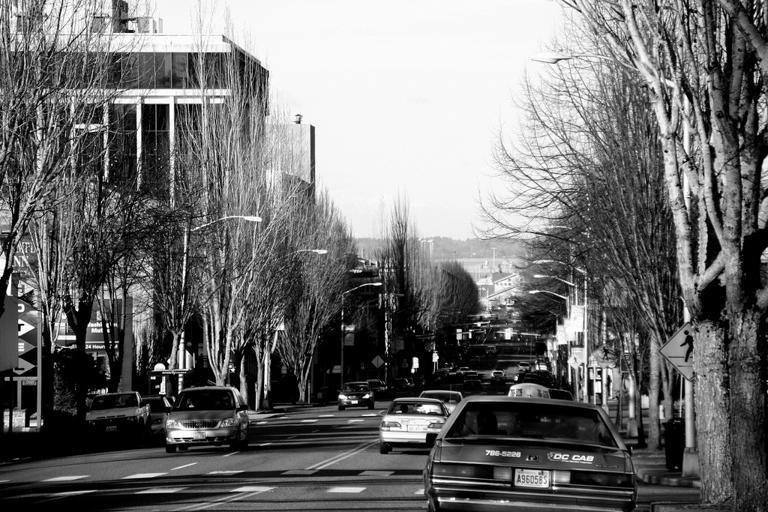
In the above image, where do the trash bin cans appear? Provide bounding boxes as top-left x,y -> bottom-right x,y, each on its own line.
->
662,417 -> 685,471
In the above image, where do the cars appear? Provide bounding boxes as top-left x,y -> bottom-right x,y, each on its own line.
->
86,384 -> 252,453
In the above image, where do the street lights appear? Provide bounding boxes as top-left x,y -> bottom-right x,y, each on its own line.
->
264,248 -> 327,410
340,282 -> 384,389
178,214 -> 264,404
528,223 -> 589,406
